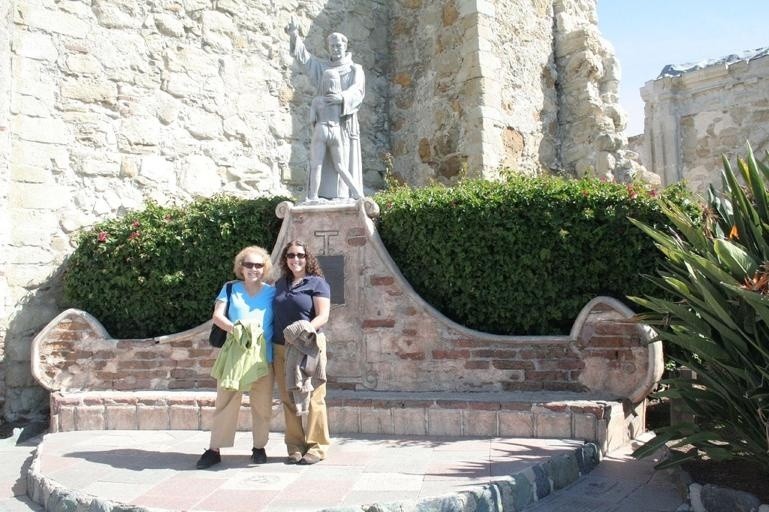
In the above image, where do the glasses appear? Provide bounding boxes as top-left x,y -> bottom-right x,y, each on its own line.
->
242,262 -> 265,268
286,253 -> 306,258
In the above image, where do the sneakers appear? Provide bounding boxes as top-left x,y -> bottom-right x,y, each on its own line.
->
251,448 -> 267,464
288,452 -> 320,464
197,448 -> 221,469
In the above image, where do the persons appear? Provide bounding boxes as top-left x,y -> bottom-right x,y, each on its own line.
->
285,14 -> 366,199
309,69 -> 362,201
273,240 -> 331,464
196,245 -> 277,469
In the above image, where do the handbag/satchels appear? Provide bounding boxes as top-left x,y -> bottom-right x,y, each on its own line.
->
209,315 -> 229,348
